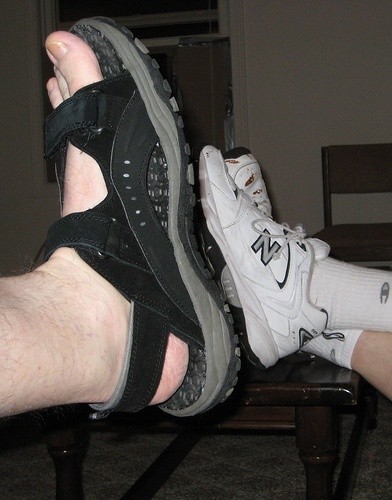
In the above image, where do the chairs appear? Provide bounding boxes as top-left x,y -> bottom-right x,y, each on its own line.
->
306,143 -> 392,270
28,159 -> 378,500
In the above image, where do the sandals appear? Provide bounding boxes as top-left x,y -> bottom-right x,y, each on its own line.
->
43,16 -> 241,418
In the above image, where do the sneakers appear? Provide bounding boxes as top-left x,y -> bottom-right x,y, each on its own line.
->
220,147 -> 271,309
199,146 -> 332,368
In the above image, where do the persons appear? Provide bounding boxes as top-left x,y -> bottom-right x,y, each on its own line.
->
199,144 -> 392,399
0,16 -> 240,417
